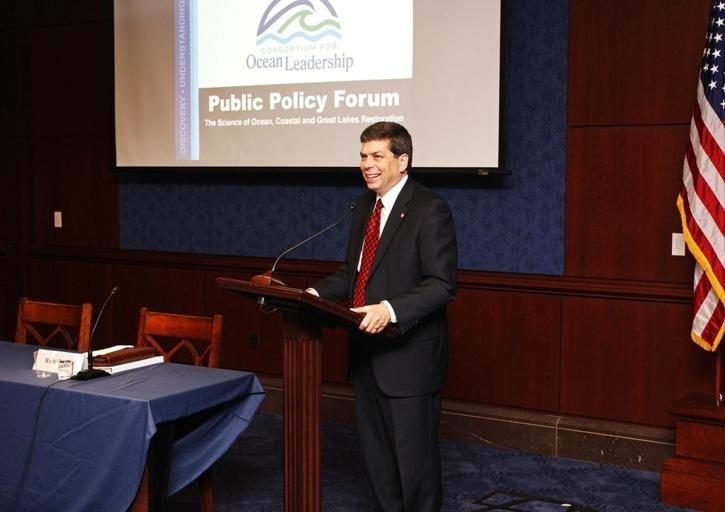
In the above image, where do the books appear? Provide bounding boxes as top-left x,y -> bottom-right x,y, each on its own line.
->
89,344 -> 158,367
93,356 -> 168,376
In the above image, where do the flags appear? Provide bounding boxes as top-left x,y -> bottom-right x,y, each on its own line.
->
674,1 -> 725,355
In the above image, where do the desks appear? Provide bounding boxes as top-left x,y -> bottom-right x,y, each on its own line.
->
0,340 -> 261,512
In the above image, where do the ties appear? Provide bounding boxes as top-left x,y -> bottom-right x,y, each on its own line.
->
351,198 -> 384,309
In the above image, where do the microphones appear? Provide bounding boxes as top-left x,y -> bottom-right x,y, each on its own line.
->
249,201 -> 358,288
70,285 -> 120,382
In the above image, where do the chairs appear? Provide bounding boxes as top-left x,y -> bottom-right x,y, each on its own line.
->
10,296 -> 95,354
134,304 -> 226,512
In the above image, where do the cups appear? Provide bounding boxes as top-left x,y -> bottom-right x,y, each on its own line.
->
33,350 -> 52,378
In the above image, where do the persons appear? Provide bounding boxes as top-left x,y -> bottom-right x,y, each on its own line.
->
304,121 -> 460,510
